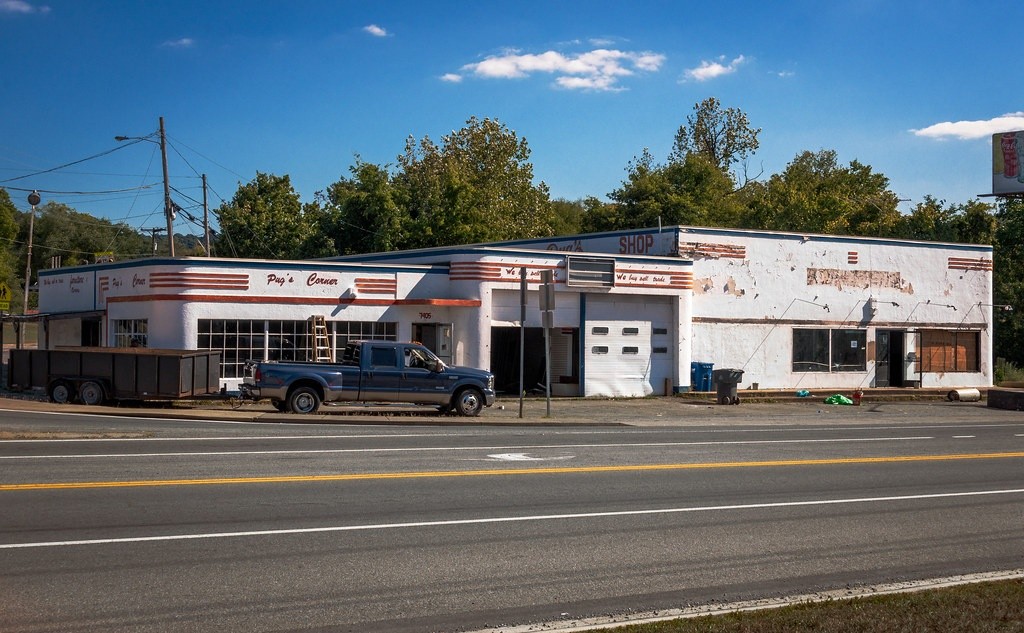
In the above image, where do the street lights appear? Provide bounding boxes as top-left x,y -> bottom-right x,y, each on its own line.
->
115,135 -> 175,258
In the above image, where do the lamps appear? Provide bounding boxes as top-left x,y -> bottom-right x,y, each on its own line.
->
929,303 -> 957,311
877,300 -> 899,307
348,287 -> 360,298
980,303 -> 1013,311
796,299 -> 830,312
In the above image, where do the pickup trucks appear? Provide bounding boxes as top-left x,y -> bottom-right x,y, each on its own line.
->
238,339 -> 496,414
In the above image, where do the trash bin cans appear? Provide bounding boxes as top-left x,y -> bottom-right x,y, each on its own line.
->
690,361 -> 714,391
712,368 -> 745,405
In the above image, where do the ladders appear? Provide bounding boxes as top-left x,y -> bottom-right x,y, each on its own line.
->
311,314 -> 333,362
